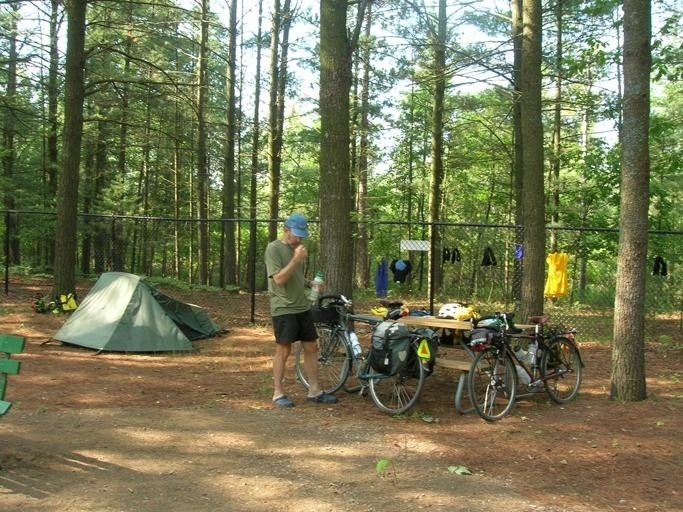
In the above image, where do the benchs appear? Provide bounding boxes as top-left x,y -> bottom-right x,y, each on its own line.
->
332,345 -> 490,414
0,335 -> 24,416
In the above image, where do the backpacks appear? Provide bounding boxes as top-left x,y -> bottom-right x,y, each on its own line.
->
409,326 -> 438,379
365,320 -> 411,378
469,318 -> 502,353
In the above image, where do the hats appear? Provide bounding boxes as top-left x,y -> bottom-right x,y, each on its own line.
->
283,212 -> 308,238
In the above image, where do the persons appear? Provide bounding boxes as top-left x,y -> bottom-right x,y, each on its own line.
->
265,215 -> 339,408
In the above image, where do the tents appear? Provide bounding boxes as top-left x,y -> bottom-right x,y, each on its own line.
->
40,272 -> 230,358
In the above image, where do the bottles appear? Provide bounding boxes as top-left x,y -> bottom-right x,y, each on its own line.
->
308,271 -> 324,301
350,331 -> 362,357
513,344 -> 526,358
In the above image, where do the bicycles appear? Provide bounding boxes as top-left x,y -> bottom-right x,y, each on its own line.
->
469,314 -> 588,422
296,293 -> 426,415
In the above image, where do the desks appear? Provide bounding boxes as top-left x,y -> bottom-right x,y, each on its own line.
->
363,313 -> 535,332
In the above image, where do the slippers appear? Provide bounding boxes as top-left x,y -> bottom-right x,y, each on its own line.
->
306,391 -> 338,405
271,394 -> 294,408
